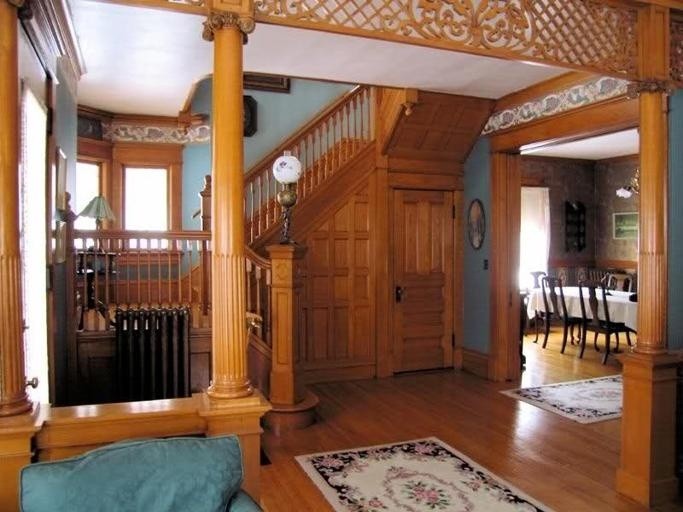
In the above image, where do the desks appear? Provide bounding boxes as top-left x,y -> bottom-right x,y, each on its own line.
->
76,249 -> 119,331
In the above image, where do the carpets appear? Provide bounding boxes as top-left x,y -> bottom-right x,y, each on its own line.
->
293,436 -> 560,512
493,371 -> 626,423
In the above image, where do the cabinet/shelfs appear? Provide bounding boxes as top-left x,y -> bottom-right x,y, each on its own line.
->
566,202 -> 586,252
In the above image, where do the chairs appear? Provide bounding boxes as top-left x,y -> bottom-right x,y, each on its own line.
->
524,266 -> 640,366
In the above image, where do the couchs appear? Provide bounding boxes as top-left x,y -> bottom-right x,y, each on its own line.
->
17,435 -> 266,510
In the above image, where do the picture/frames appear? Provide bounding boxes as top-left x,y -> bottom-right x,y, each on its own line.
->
242,74 -> 292,94
611,212 -> 638,240
468,198 -> 485,250
55,148 -> 67,212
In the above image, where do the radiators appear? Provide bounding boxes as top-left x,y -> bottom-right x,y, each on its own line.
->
115,308 -> 191,400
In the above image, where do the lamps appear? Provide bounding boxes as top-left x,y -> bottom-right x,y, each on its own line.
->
272,148 -> 301,245
615,170 -> 641,200
79,194 -> 113,250
